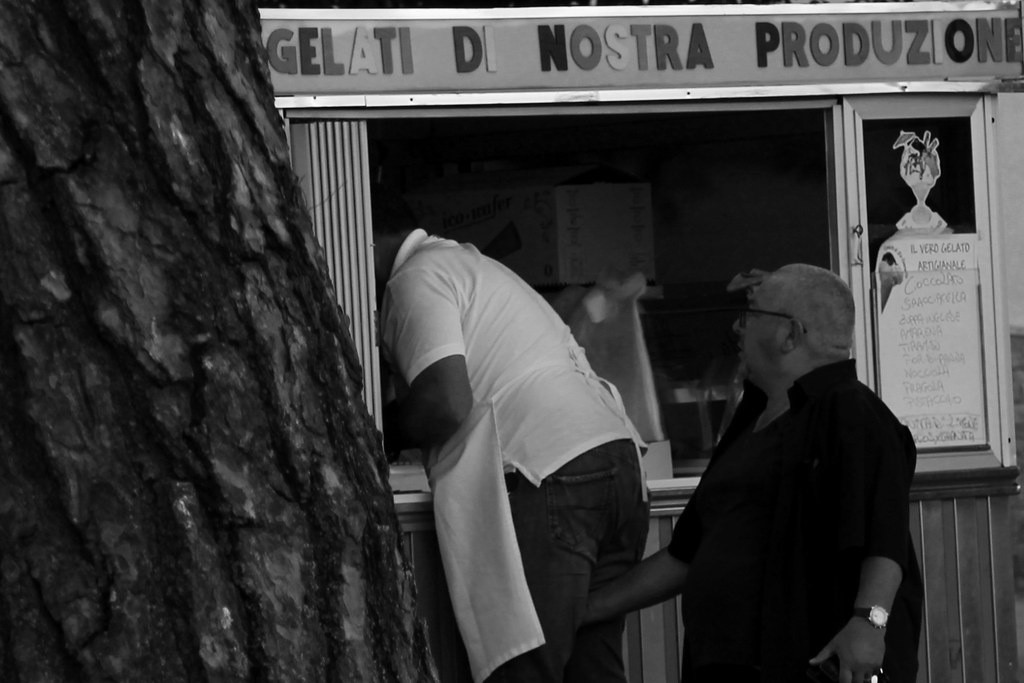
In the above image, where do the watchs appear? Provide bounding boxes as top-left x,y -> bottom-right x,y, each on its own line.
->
849,605 -> 889,629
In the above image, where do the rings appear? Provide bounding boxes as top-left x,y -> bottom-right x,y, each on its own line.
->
879,668 -> 883,674
863,675 -> 879,683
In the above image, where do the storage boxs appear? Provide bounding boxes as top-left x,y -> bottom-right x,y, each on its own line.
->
399,176 -> 657,290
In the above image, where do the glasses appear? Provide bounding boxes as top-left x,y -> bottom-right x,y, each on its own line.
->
739,306 -> 808,334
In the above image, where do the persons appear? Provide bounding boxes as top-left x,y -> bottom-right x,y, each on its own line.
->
581,265 -> 924,683
371,183 -> 651,683
